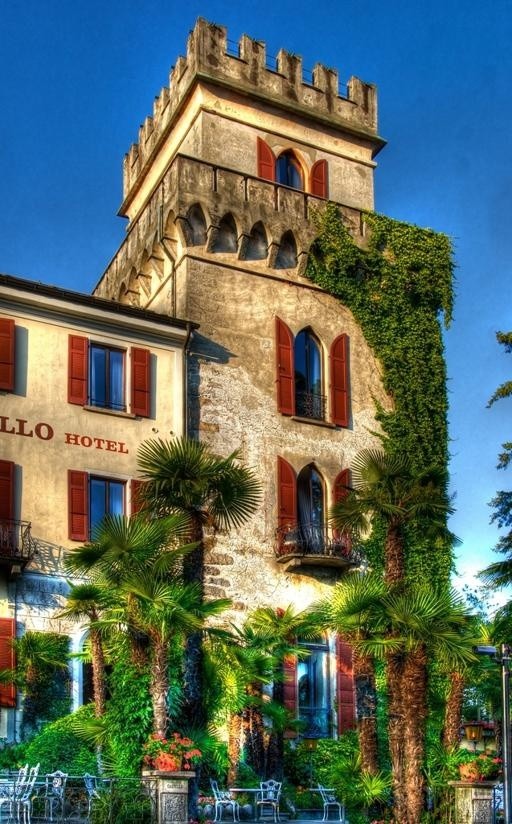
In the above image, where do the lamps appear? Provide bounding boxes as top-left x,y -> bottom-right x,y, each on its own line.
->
462,720 -> 486,757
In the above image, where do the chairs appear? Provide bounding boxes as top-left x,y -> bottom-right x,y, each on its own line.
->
208,778 -> 240,823
0,762 -> 115,824
258,780 -> 284,823
315,783 -> 343,822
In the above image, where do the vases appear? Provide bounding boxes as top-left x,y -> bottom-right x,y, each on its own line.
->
460,763 -> 480,781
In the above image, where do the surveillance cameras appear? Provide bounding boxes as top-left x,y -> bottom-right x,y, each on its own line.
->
472,645 -> 497,655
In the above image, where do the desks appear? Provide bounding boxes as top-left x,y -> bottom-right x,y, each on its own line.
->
312,787 -> 336,794
228,785 -> 266,821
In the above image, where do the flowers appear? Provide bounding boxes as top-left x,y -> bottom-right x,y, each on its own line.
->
143,735 -> 201,772
453,741 -> 503,785
